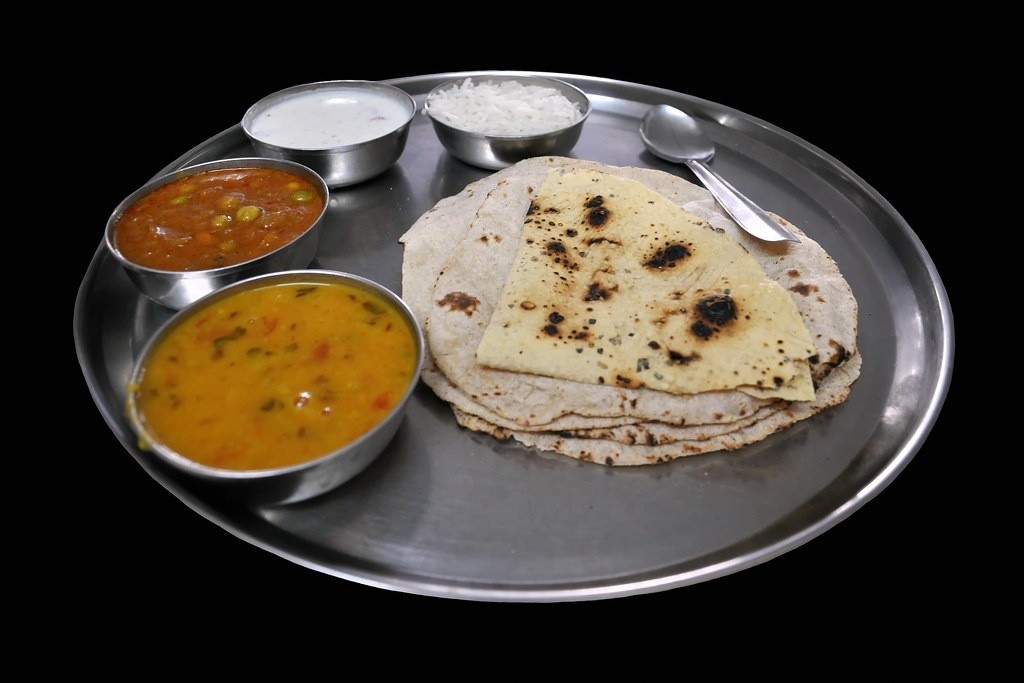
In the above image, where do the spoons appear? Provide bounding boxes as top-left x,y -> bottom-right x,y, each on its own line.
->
638,103 -> 802,245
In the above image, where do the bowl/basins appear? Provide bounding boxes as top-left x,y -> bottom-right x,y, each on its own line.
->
424,74 -> 593,173
126,267 -> 425,509
104,156 -> 329,310
241,80 -> 418,191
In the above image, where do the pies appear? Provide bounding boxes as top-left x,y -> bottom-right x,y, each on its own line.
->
398,157 -> 864,465
